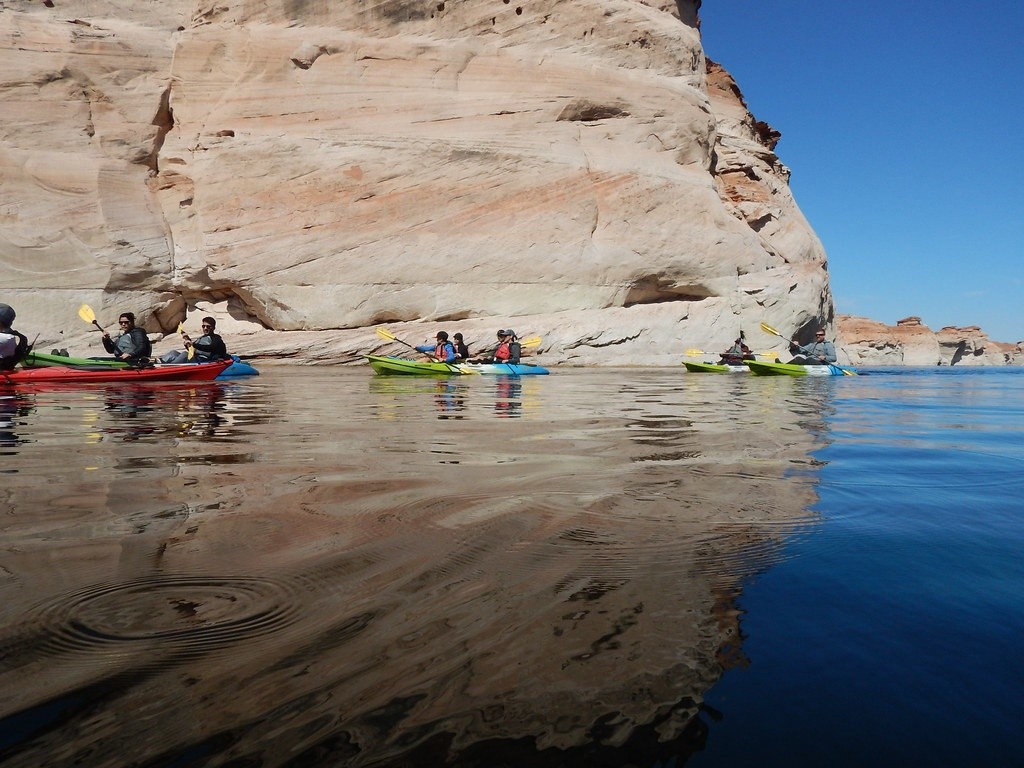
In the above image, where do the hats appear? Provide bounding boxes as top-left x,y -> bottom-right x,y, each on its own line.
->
501,329 -> 516,335
453,333 -> 463,341
432,331 -> 448,339
497,330 -> 505,335
0,303 -> 16,325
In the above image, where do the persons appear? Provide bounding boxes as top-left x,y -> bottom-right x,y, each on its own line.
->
0,303 -> 28,372
412,329 -> 522,367
716,328 -> 836,367
50,313 -> 152,365
154,316 -> 226,365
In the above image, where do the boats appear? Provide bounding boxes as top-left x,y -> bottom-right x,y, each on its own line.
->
19,351 -> 263,378
681,360 -> 751,373
1,356 -> 232,385
362,353 -> 551,376
742,359 -> 858,378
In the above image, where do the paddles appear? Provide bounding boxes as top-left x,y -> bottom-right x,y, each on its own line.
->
376,326 -> 483,375
760,322 -> 859,378
178,320 -> 195,360
77,303 -> 137,369
686,349 -> 778,360
473,336 -> 542,356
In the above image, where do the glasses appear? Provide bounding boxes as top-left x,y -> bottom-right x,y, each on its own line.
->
815,334 -> 825,337
202,325 -> 212,329
119,321 -> 129,325
454,338 -> 460,340
